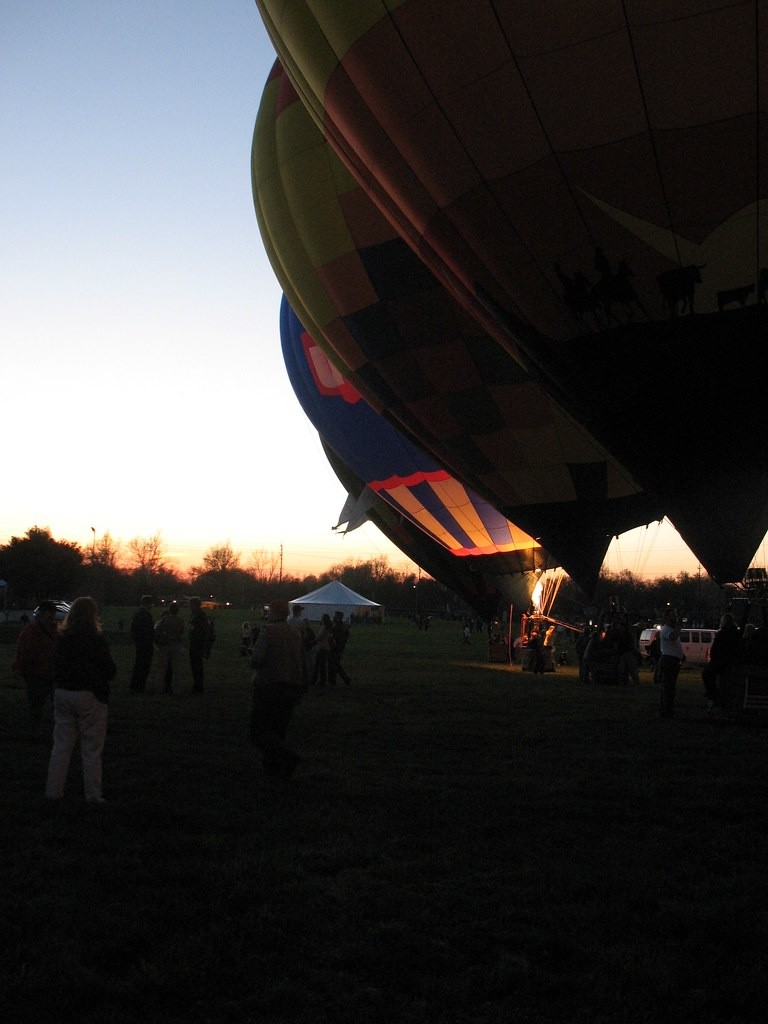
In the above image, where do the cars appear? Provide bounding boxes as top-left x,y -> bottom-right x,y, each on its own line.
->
33,600 -> 74,627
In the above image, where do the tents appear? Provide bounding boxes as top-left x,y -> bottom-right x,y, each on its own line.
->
288,581 -> 385,624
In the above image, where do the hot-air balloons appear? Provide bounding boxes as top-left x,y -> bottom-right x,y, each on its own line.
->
253,0 -> 768,730
252,55 -> 666,670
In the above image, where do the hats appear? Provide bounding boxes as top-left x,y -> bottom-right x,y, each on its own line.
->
270,598 -> 289,618
293,605 -> 304,614
37,600 -> 62,612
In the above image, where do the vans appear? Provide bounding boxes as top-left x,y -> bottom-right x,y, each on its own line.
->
678,628 -> 719,668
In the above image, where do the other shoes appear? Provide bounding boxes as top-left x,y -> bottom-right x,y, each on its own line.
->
87,795 -> 108,805
707,698 -> 719,716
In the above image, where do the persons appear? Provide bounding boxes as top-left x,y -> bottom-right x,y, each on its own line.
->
44,598 -> 119,807
12,596 -> 768,780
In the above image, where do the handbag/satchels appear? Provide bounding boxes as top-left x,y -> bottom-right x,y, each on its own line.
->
318,635 -> 337,650
